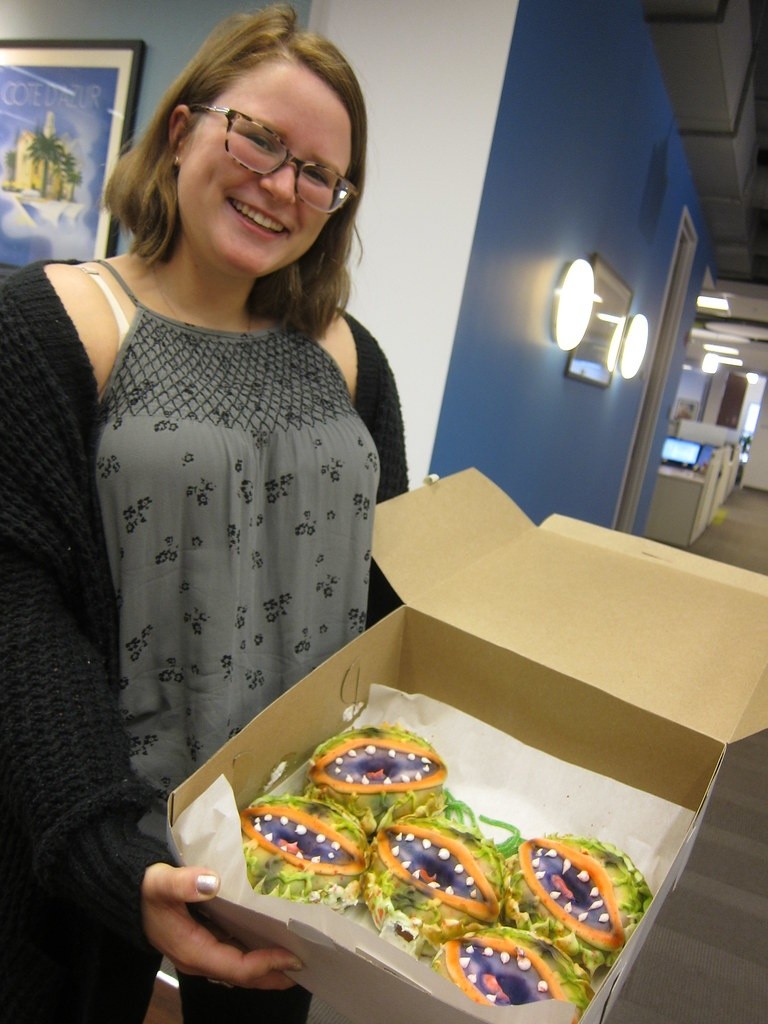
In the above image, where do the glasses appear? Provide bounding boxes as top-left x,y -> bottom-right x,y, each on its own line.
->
187,104 -> 360,214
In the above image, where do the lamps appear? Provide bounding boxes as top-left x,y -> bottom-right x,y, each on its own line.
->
551,257 -> 596,350
618,313 -> 648,380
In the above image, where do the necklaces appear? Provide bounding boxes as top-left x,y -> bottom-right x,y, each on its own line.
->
158,264 -> 254,331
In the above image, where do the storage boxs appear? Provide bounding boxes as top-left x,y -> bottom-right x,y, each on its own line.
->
168,465 -> 768,1024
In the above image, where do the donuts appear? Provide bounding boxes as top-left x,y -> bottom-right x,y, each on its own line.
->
233,723 -> 655,1024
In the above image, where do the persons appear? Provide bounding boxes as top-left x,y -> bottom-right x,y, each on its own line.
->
1,0 -> 409,1023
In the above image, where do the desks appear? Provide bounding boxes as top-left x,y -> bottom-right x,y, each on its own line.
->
643,422 -> 740,546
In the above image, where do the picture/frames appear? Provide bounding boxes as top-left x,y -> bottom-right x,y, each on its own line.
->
1,37 -> 146,270
566,252 -> 635,389
